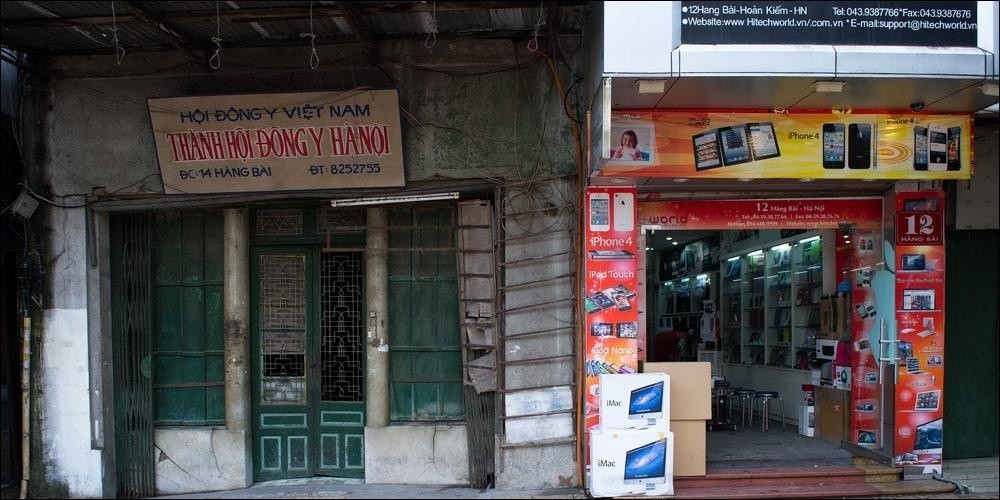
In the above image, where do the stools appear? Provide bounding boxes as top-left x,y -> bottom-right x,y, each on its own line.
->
717,386 -> 785,433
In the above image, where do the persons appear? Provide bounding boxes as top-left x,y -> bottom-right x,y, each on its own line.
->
613,130 -> 643,160
616,295 -> 628,307
675,322 -> 698,360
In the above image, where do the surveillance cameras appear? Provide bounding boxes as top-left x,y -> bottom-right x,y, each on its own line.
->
910,102 -> 926,112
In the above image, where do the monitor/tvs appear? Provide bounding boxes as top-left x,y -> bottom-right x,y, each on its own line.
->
912,418 -> 943,461
623,437 -> 667,489
628,380 -> 665,429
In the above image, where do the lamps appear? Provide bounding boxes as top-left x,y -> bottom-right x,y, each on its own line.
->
977,82 -> 999,97
810,81 -> 846,93
634,79 -> 667,94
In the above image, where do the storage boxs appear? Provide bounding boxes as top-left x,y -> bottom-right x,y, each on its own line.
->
589,361 -> 712,498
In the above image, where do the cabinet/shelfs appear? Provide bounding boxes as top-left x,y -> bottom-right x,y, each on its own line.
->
719,230 -> 834,428
658,271 -> 719,358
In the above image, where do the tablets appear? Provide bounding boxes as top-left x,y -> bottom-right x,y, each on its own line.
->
692,122 -> 781,170
609,123 -> 655,166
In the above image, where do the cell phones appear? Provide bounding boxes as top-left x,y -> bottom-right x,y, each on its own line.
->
585,192 -> 639,378
823,122 -> 877,170
854,239 -> 878,411
913,121 -> 961,172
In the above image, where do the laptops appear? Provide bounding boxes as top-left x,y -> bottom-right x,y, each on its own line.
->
857,430 -> 881,449
896,254 -> 942,413
